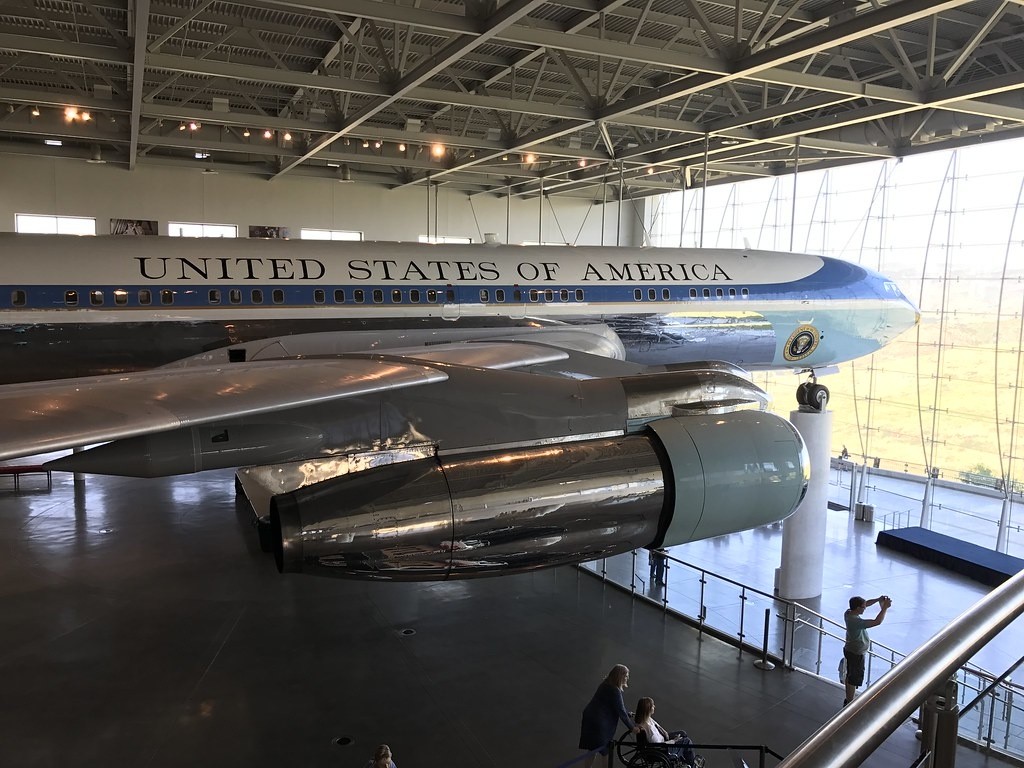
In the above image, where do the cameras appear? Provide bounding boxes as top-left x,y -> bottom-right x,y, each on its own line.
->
879,598 -> 885,606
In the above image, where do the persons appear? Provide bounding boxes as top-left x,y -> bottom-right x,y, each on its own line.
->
364,744 -> 397,768
649,548 -> 665,586
578,663 -> 640,768
843,596 -> 891,707
634,697 -> 699,768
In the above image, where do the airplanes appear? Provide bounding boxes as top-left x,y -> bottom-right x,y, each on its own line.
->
0,231 -> 922,585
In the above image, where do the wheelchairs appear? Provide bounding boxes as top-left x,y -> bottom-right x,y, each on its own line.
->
618,712 -> 707,768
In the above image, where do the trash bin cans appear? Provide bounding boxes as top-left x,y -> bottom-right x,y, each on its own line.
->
862,504 -> 874,522
854,502 -> 866,522
